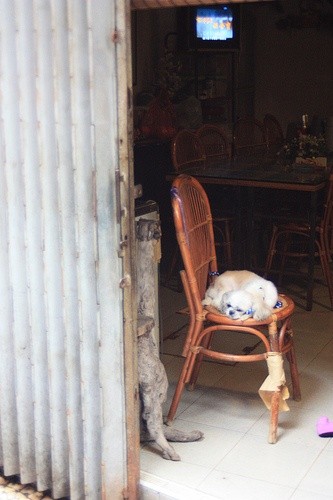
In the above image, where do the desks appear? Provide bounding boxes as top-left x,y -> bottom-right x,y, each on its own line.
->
164,146 -> 328,311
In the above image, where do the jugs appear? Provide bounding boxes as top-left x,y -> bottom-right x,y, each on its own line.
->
296,113 -> 311,147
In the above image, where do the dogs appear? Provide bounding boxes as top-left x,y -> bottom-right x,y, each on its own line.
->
135,217 -> 206,461
201,269 -> 279,321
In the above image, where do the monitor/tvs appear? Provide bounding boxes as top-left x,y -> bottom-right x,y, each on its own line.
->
183,5 -> 240,51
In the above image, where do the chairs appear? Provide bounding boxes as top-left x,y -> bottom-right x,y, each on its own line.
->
167,115 -> 333,444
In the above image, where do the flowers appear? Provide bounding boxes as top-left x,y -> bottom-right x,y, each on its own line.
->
152,50 -> 186,101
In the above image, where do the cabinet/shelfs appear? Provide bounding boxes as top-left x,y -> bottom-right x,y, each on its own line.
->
164,30 -> 255,157
